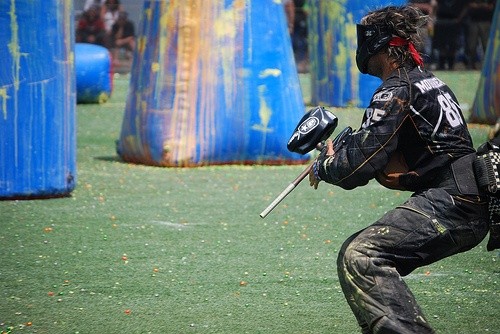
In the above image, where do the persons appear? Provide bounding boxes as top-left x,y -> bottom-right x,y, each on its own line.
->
76,0 -> 137,52
410,0 -> 496,71
309,4 -> 490,334
285,0 -> 309,73
